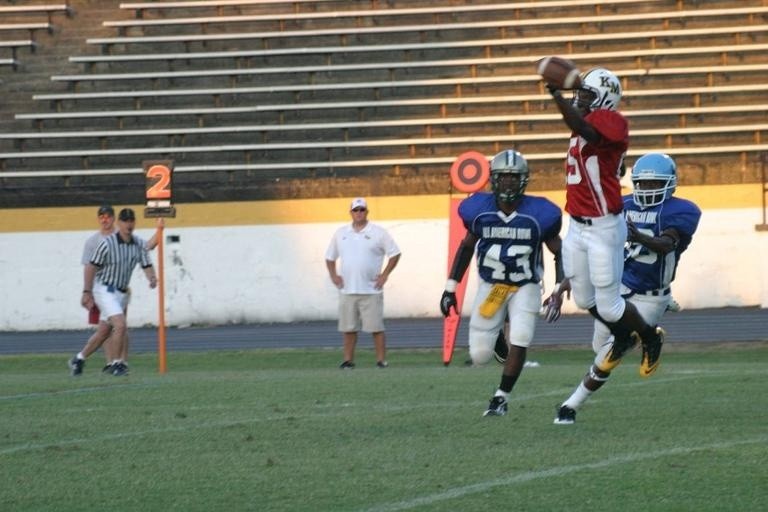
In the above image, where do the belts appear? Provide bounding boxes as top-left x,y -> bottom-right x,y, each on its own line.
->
622,282 -> 673,298
568,211 -> 594,229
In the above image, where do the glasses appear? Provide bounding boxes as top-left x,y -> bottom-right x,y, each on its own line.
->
351,209 -> 365,212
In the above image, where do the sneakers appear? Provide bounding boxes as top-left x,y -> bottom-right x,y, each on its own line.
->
482,396 -> 508,418
377,361 -> 388,367
68,355 -> 85,376
639,326 -> 666,377
600,331 -> 638,371
551,404 -> 577,425
493,330 -> 508,363
101,362 -> 131,377
340,360 -> 355,369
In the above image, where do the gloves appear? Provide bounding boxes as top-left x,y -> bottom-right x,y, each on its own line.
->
543,292 -> 562,323
440,290 -> 459,318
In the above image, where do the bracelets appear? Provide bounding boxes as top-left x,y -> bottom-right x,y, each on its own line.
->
83,290 -> 91,293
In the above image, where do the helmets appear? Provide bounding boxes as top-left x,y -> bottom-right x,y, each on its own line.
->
569,67 -> 622,112
630,153 -> 677,207
489,150 -> 529,202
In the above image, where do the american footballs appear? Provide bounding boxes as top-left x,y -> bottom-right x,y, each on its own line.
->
539,57 -> 581,89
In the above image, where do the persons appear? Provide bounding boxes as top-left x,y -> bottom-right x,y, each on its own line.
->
324,198 -> 402,368
440,149 -> 563,417
535,55 -> 666,377
81,205 -> 164,375
552,152 -> 702,425
68,208 -> 157,376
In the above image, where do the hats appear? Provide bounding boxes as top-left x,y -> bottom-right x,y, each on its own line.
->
350,198 -> 366,209
119,208 -> 134,220
97,206 -> 114,217
